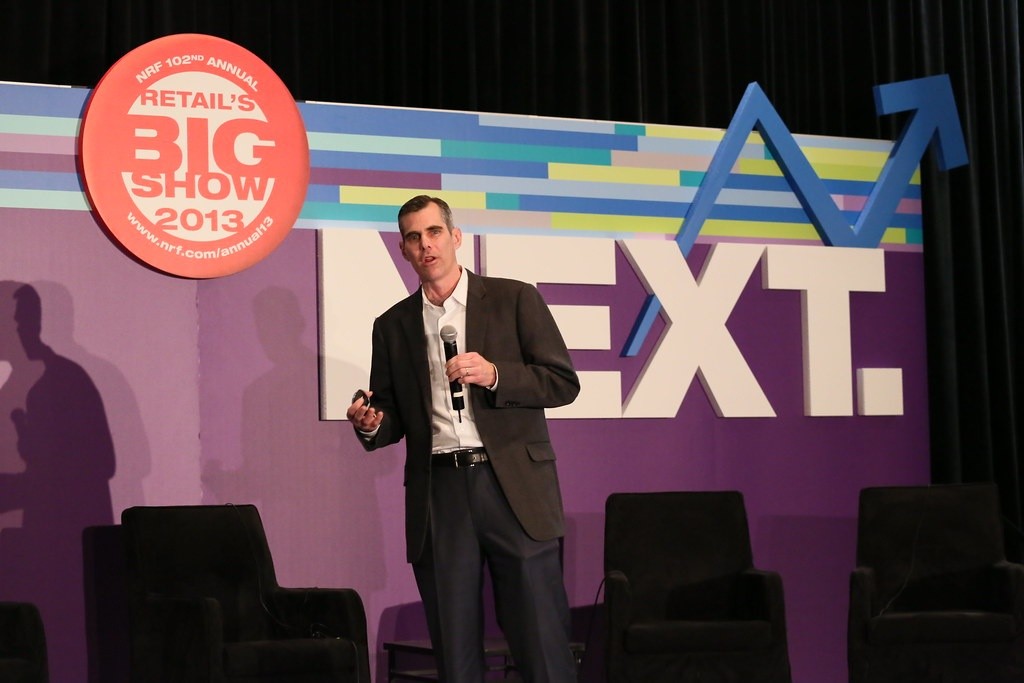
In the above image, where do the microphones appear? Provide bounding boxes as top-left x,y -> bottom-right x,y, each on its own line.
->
440,325 -> 465,424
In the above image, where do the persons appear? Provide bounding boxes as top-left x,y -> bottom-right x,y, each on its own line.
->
346,195 -> 580,683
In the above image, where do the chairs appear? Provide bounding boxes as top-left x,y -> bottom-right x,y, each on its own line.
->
0,602 -> 49,683
122,505 -> 372,683
603,490 -> 794,683
847,485 -> 1024,683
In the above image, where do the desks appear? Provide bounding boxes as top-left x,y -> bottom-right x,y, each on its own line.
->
382,641 -> 586,683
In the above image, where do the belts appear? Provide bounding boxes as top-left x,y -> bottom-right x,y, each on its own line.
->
431,449 -> 488,468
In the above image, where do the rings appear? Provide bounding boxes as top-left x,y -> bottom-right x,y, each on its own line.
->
466,368 -> 469,375
